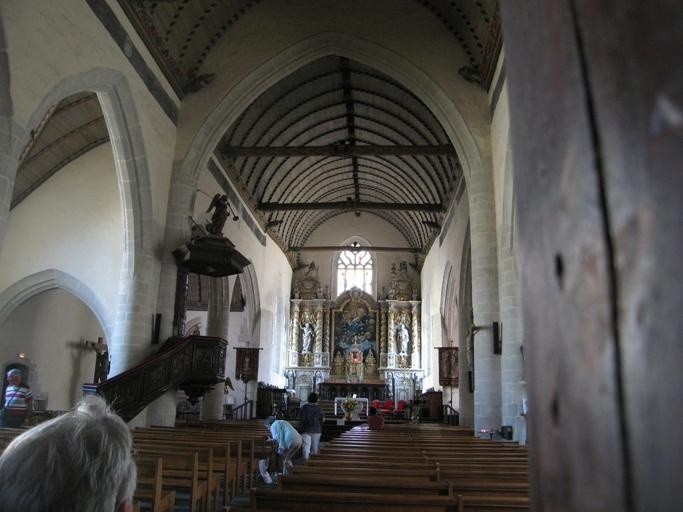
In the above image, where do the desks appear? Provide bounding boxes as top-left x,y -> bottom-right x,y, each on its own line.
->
334,398 -> 369,416
256,388 -> 286,418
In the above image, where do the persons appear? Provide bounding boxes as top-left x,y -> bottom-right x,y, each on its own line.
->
368,406 -> 385,430
264,416 -> 303,476
93,336 -> 109,383
397,324 -> 409,355
1,398 -> 140,511
298,392 -> 326,465
2,369 -> 32,430
299,320 -> 315,354
210,194 -> 230,234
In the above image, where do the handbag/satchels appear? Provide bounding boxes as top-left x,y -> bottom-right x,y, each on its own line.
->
0,409 -> 6,424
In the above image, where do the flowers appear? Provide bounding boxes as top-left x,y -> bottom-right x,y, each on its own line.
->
339,399 -> 359,413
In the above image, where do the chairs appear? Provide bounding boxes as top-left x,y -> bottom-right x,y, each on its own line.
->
393,400 -> 407,423
372,400 -> 379,408
380,400 -> 394,423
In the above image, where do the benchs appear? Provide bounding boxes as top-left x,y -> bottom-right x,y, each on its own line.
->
249,423 -> 530,512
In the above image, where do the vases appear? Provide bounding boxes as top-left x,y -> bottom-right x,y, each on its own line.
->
346,412 -> 353,422
